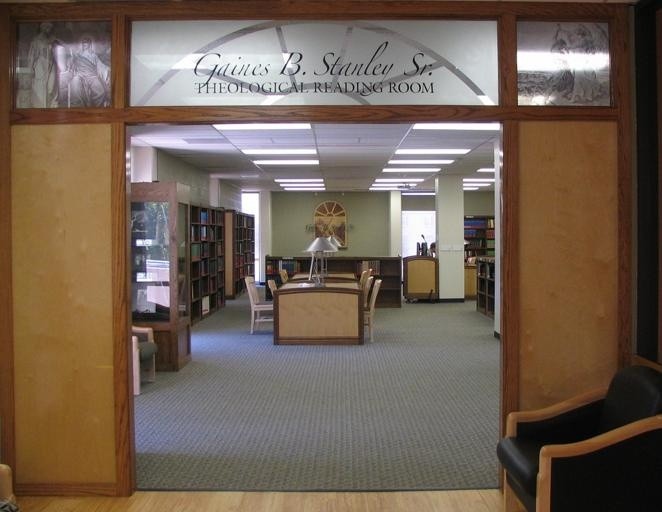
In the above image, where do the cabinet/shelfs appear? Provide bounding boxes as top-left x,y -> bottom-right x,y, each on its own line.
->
131,180 -> 192,371
265,254 -> 402,308
475,259 -> 495,319
464,214 -> 495,262
224,208 -> 255,299
189,201 -> 226,326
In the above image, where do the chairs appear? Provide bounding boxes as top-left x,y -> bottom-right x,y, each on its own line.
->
130,325 -> 158,395
359,268 -> 382,343
244,269 -> 289,334
497,354 -> 662,512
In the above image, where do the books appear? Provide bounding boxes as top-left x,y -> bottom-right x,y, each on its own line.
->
190,205 -> 254,318
357,261 -> 384,275
464,217 -> 494,317
267,260 -> 301,297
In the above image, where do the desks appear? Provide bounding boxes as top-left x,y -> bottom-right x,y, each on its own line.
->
272,271 -> 364,344
464,263 -> 479,300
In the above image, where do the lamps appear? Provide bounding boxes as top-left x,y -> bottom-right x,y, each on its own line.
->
302,235 -> 342,287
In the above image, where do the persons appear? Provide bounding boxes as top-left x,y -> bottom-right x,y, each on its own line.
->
27,22 -> 112,108
